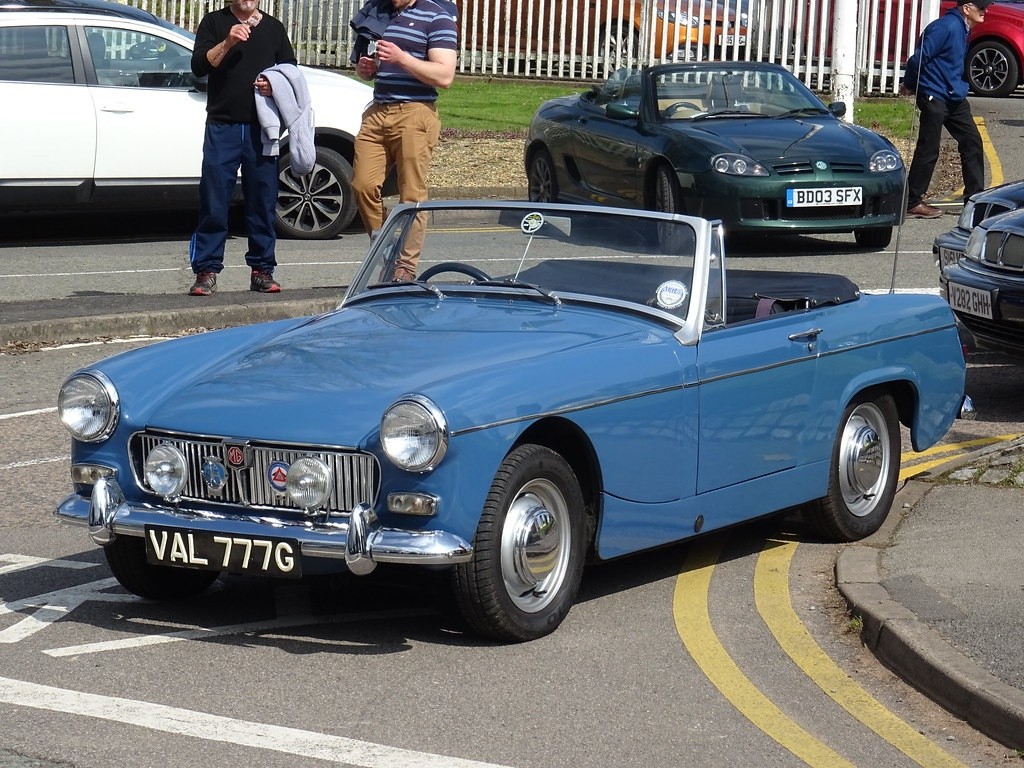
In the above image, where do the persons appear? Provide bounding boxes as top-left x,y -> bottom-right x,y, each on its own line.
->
189,0 -> 298,293
350,0 -> 458,282
903,0 -> 994,218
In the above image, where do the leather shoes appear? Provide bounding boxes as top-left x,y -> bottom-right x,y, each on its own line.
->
906,203 -> 943,219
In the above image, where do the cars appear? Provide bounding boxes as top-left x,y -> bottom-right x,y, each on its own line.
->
770,0 -> 1024,97
450,0 -> 756,78
940,207 -> 1024,363
524,60 -> 909,247
53,200 -> 978,642
933,179 -> 1024,291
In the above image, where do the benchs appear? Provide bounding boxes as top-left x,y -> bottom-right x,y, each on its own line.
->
373,254 -> 858,334
580,91 -> 764,119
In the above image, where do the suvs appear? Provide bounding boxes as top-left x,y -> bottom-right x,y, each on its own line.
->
0,0 -> 377,240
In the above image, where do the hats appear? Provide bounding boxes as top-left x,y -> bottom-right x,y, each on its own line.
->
957,0 -> 996,9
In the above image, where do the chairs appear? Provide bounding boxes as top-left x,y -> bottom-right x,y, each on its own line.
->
620,76 -> 643,112
706,77 -> 751,115
87,33 -> 117,85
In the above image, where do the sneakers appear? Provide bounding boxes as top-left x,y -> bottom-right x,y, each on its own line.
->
250,269 -> 282,293
188,272 -> 217,295
379,265 -> 415,284
